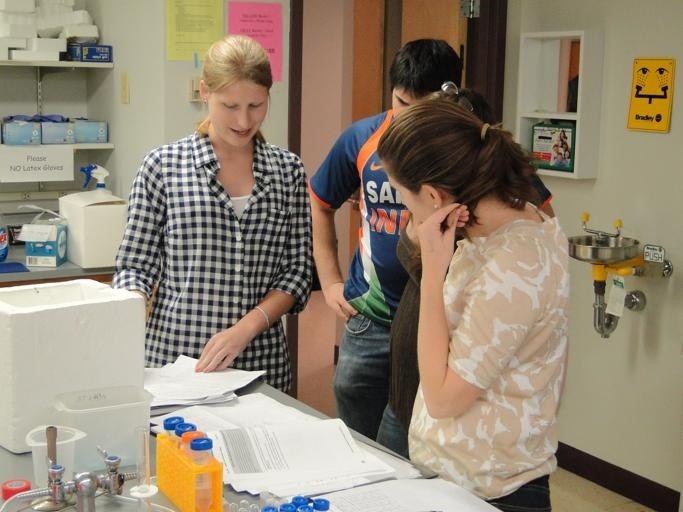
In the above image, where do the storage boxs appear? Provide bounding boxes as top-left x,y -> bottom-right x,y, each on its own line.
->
59,191 -> 129,269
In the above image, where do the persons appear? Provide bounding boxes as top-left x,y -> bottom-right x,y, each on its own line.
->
374,95 -> 569,512
551,129 -> 570,168
305,35 -> 465,470
108,35 -> 312,400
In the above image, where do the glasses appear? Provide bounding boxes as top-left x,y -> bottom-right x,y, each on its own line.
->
442,82 -> 474,113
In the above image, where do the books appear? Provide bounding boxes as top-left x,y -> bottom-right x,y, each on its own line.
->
142,351 -> 398,502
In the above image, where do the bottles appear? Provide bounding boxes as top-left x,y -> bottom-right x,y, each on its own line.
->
156,416 -> 224,512
230,491 -> 330,512
135,425 -> 150,493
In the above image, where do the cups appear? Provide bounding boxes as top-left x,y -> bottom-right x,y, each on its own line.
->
25,425 -> 87,488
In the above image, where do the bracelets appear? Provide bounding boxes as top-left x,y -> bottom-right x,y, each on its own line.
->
256,305 -> 271,332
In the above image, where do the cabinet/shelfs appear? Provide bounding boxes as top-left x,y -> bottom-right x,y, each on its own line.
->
516,30 -> 603,182
0,59 -> 129,287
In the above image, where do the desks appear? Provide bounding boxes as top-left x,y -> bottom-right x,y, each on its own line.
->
0,380 -> 502,512
